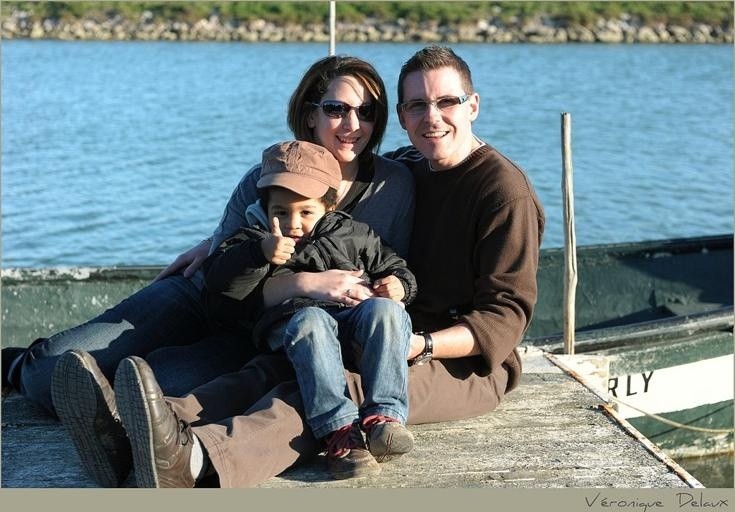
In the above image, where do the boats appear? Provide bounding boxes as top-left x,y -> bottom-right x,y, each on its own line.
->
0,227 -> 734,485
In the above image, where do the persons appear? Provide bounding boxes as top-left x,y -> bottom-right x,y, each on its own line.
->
47,45 -> 547,488
1,52 -> 419,421
201,140 -> 419,478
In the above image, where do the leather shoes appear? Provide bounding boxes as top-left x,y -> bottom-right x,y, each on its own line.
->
114,355 -> 197,489
50,348 -> 134,488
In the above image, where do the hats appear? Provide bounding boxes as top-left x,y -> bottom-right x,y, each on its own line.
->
256,140 -> 342,199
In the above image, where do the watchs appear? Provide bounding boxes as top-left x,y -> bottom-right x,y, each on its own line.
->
406,329 -> 435,367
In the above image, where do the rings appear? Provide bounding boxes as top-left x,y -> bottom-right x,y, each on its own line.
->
345,288 -> 353,297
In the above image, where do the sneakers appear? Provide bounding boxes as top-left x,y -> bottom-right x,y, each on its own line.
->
325,424 -> 382,481
363,415 -> 415,464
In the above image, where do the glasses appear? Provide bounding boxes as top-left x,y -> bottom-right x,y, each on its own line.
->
398,94 -> 469,116
312,99 -> 377,122
